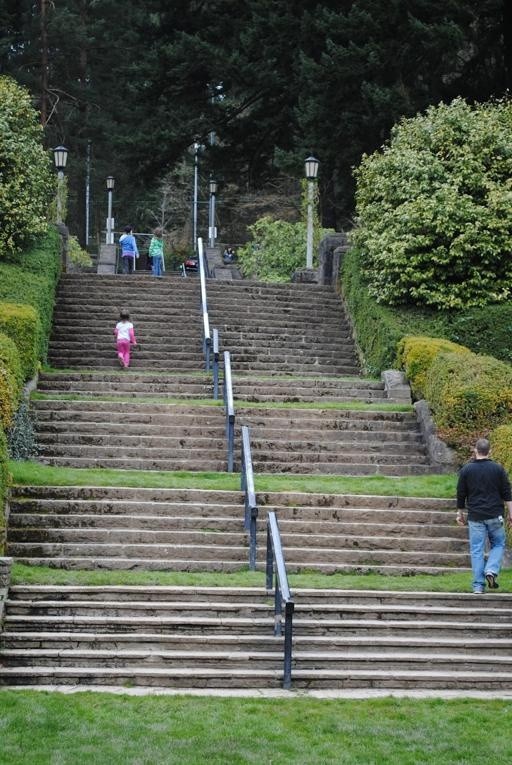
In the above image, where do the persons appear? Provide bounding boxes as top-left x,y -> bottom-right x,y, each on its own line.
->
118,225 -> 139,273
456,438 -> 511,594
113,308 -> 136,372
147,226 -> 167,274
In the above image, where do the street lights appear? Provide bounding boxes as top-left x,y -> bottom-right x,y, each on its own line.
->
191,141 -> 201,252
304,156 -> 320,268
52,143 -> 71,224
104,175 -> 117,242
85,139 -> 92,245
208,180 -> 218,248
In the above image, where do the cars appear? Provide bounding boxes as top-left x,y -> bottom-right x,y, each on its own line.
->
185,255 -> 199,271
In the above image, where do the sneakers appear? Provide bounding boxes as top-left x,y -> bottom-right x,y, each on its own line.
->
485,573 -> 498,588
